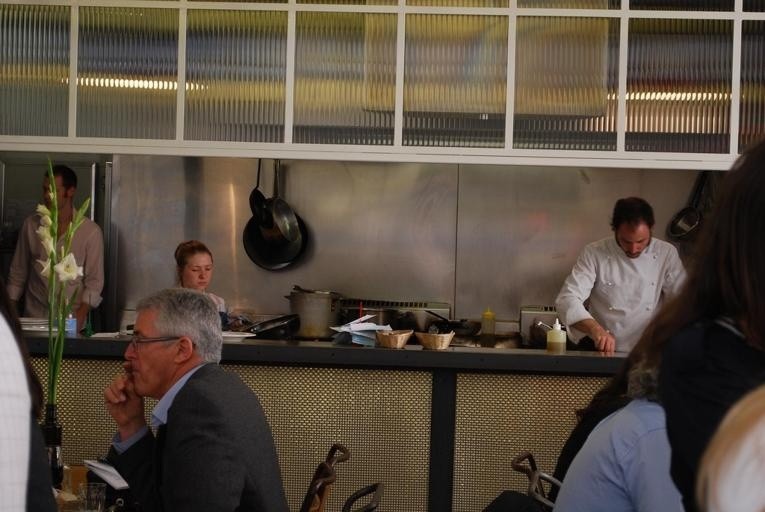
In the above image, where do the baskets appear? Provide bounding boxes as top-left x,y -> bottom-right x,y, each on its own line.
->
375,329 -> 413,349
414,330 -> 456,350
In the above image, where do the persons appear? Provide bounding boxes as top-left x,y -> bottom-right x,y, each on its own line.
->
5,163 -> 105,334
68,285 -> 291,511
172,238 -> 228,313
0,283 -> 47,511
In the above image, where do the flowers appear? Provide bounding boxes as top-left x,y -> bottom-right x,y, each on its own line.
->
36,152 -> 90,403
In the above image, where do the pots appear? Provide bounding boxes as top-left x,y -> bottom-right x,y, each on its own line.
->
284,285 -> 342,338
338,307 -> 414,330
243,158 -> 307,271
241,314 -> 301,337
425,309 -> 481,335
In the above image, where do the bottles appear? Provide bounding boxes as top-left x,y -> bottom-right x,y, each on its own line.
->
481,305 -> 496,336
546,317 -> 567,351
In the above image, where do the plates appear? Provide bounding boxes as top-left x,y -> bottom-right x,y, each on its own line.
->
222,331 -> 256,342
15,317 -> 72,336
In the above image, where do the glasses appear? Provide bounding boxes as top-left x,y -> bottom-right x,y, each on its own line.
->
130,334 -> 179,349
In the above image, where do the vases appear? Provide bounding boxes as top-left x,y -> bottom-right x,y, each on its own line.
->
39,404 -> 62,490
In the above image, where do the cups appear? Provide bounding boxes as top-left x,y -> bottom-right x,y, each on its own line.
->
79,483 -> 107,512
